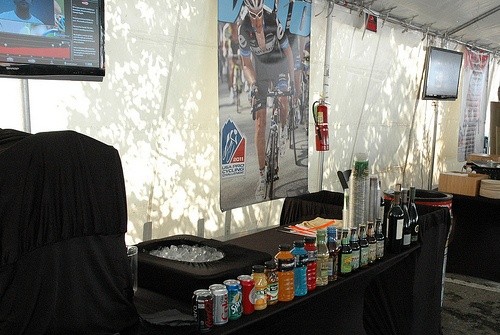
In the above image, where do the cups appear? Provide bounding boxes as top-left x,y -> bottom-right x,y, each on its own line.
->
125,245 -> 138,293
342,151 -> 381,229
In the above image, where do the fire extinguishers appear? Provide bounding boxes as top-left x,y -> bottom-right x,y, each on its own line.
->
315,96 -> 330,152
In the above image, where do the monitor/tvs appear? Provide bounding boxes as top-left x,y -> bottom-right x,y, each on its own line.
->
0,0 -> 105,81
422,47 -> 463,101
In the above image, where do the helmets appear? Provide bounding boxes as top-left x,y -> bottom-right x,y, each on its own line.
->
244,0 -> 263,10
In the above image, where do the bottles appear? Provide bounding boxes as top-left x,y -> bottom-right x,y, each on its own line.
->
290,241 -> 308,297
385,183 -> 420,249
264,260 -> 279,305
327,217 -> 384,282
251,265 -> 267,310
316,229 -> 329,285
304,236 -> 317,290
379,181 -> 384,225
274,243 -> 295,301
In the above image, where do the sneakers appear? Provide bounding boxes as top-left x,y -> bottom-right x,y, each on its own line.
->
280,133 -> 287,158
255,179 -> 266,202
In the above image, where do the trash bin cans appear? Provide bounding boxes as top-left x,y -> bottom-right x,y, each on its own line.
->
381,188 -> 456,334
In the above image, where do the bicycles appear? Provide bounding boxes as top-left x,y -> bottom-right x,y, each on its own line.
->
218,55 -> 309,200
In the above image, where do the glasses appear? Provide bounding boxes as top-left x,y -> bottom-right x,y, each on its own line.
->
249,10 -> 263,19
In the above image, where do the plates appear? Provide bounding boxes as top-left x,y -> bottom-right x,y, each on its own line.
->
480,179 -> 500,199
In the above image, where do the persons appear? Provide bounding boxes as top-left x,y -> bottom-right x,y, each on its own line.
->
221,0 -> 311,203
0,0 -> 43,24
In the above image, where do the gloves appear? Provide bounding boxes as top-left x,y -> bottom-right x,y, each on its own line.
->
251,82 -> 258,99
